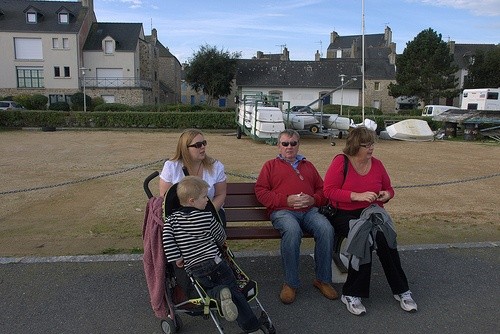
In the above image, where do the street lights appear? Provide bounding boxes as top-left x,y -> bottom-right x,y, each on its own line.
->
338,74 -> 347,116
80,67 -> 90,112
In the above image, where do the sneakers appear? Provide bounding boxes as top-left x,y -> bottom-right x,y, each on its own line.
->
313,277 -> 338,299
341,294 -> 366,315
280,282 -> 297,304
393,289 -> 417,312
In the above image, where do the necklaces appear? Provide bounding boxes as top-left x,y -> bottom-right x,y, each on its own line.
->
196,161 -> 202,175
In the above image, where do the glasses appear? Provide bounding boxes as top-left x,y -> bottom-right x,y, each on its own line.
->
188,140 -> 207,148
359,143 -> 375,148
279,141 -> 299,146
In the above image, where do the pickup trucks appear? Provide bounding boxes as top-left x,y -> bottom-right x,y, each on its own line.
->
282,106 -> 330,133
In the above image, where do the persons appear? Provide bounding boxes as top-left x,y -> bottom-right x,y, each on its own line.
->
159,128 -> 227,237
162,175 -> 265,334
254,130 -> 339,304
322,126 -> 418,315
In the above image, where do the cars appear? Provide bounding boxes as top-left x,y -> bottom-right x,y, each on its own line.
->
0,101 -> 27,111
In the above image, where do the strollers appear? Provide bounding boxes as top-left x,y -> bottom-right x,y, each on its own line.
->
143,166 -> 276,334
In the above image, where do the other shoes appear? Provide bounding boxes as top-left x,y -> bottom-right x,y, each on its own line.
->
220,287 -> 238,321
249,328 -> 264,334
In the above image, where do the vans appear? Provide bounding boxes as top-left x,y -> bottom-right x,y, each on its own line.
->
421,88 -> 500,117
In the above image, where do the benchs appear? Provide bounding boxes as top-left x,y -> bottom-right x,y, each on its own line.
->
214,182 -> 350,274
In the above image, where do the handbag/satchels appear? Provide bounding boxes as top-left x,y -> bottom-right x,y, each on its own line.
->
320,204 -> 337,223
